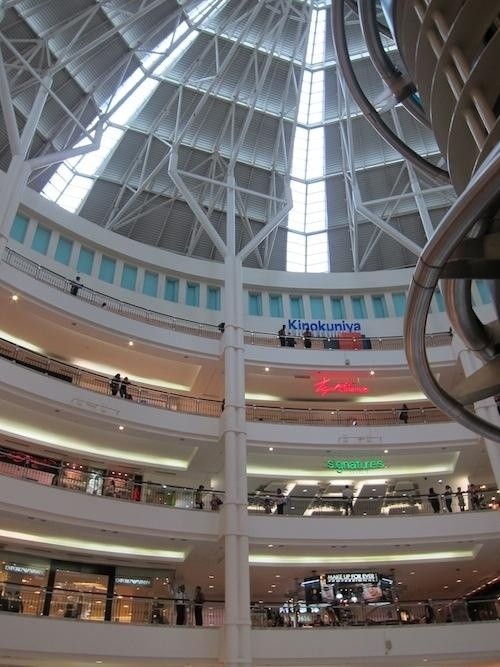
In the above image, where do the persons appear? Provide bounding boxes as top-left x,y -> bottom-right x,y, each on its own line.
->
327,601 -> 344,627
278,324 -> 288,347
488,497 -> 499,509
427,487 -> 440,512
69,275 -> 83,295
193,484 -> 204,509
454,486 -> 466,511
274,488 -> 284,514
120,376 -> 130,398
423,599 -> 433,623
322,575 -> 332,600
303,327 -> 313,349
50,469 -> 67,487
110,372 -> 119,395
4,592 -> 14,611
106,480 -> 117,497
399,403 -> 408,423
192,585 -> 205,625
285,332 -> 298,347
273,616 -> 292,628
440,484 -> 452,511
342,484 -> 354,515
10,591 -> 23,614
173,584 -> 188,626
466,484 -> 483,511
